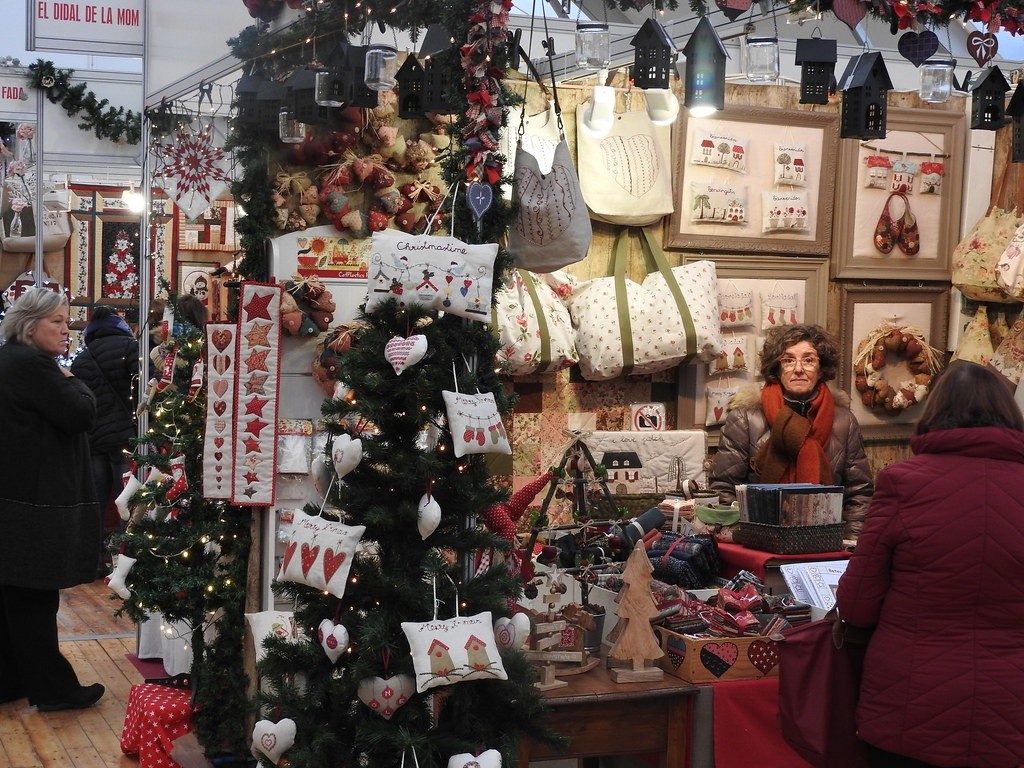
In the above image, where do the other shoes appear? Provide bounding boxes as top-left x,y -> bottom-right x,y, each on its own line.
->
40,682 -> 103,712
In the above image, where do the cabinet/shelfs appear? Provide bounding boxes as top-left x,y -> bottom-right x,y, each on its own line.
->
61,181 -> 178,367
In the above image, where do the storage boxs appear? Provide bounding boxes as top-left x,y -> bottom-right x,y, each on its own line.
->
650,599 -> 841,683
514,561 -> 574,617
573,573 -> 721,647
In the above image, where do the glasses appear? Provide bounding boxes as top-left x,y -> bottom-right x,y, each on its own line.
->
776,357 -> 823,371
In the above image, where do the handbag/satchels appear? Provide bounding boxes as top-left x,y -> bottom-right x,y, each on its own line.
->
400,570 -> 508,692
442,353 -> 511,458
133,411 -> 156,437
688,119 -> 809,234
364,179 -> 500,325
506,136 -> 593,275
770,603 -> 867,768
275,472 -> 366,599
568,226 -> 720,381
577,96 -> 673,226
950,146 -> 1024,394
483,264 -> 578,378
701,279 -> 798,426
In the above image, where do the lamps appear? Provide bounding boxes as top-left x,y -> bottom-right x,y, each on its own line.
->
643,86 -> 679,126
582,85 -> 615,132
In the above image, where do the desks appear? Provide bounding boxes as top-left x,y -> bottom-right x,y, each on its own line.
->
715,541 -> 853,596
513,642 -> 699,768
692,677 -> 815,768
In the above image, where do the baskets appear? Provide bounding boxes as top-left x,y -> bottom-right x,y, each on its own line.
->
734,519 -> 847,556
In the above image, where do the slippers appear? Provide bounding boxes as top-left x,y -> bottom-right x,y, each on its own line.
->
896,194 -> 920,256
874,194 -> 906,254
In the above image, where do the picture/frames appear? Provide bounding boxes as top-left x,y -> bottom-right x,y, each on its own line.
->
663,102 -> 840,256
678,251 -> 831,448
830,106 -> 966,281
840,286 -> 951,442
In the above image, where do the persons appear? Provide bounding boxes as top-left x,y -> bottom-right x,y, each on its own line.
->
0,288 -> 112,713
71,295 -> 205,518
709,324 -> 875,552
834,359 -> 1024,768
